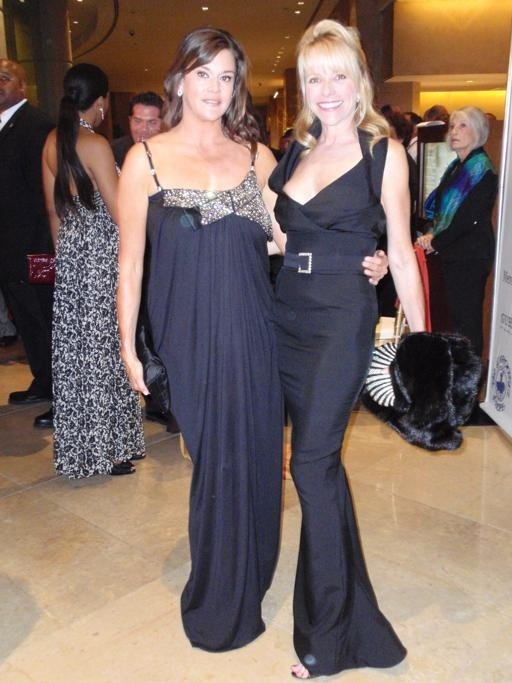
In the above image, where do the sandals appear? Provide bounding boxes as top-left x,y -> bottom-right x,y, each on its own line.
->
110,451 -> 146,475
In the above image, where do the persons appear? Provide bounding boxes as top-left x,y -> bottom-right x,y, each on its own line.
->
274,129 -> 294,161
267,21 -> 480,677
115,29 -> 388,653
2,61 -> 180,480
380,99 -> 499,354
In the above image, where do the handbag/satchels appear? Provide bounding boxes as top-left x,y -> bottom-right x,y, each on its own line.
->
136,327 -> 171,414
28,253 -> 56,282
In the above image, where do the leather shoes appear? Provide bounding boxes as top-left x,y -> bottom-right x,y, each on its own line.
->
8,390 -> 48,405
34,410 -> 53,428
